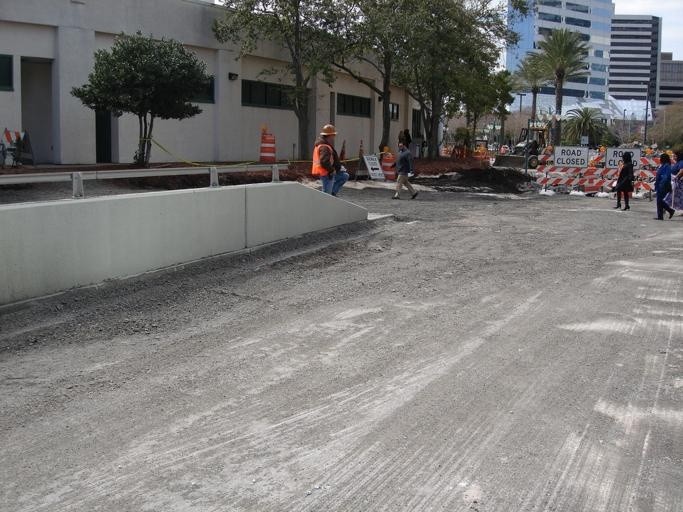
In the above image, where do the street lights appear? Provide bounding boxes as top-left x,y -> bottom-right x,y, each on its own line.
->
641,81 -> 656,145
516,92 -> 526,113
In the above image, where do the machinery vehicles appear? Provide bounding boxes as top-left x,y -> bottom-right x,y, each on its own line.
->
491,128 -> 551,169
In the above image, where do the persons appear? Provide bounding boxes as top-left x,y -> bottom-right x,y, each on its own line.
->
653,152 -> 675,220
399,128 -> 411,149
610,151 -> 634,211
311,122 -> 350,196
661,149 -> 682,217
389,141 -> 418,201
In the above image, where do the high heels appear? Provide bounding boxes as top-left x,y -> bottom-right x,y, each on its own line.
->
613,205 -> 630,211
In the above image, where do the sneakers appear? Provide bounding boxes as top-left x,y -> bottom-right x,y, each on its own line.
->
653,210 -> 675,220
392,193 -> 418,199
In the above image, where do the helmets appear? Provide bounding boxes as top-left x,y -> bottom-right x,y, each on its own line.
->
320,124 -> 338,136
